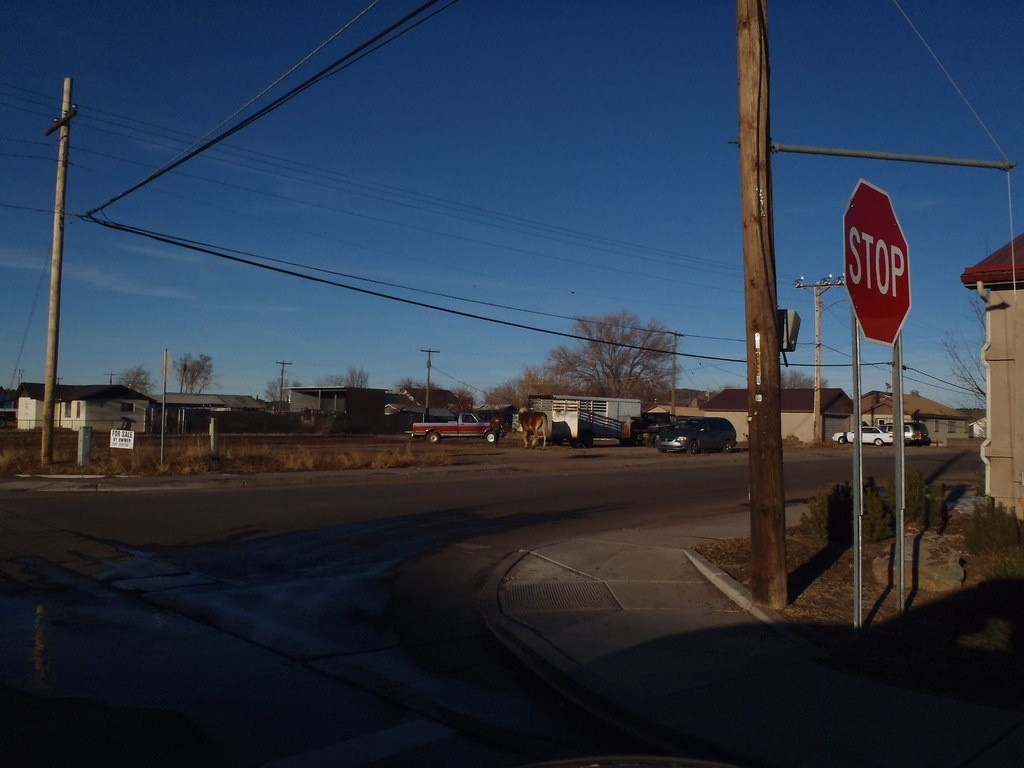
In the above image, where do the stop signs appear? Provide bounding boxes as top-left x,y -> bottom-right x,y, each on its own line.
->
843,176 -> 911,348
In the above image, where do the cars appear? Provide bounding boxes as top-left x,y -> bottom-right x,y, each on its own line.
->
656,417 -> 737,454
833,427 -> 894,446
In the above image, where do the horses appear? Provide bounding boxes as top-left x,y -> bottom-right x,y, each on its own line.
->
518,407 -> 549,450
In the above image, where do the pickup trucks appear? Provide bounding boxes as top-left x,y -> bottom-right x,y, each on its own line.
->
404,412 -> 506,444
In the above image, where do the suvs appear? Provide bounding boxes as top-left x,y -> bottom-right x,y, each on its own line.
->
880,423 -> 931,446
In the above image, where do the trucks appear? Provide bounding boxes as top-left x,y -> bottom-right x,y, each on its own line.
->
620,412 -> 679,446
